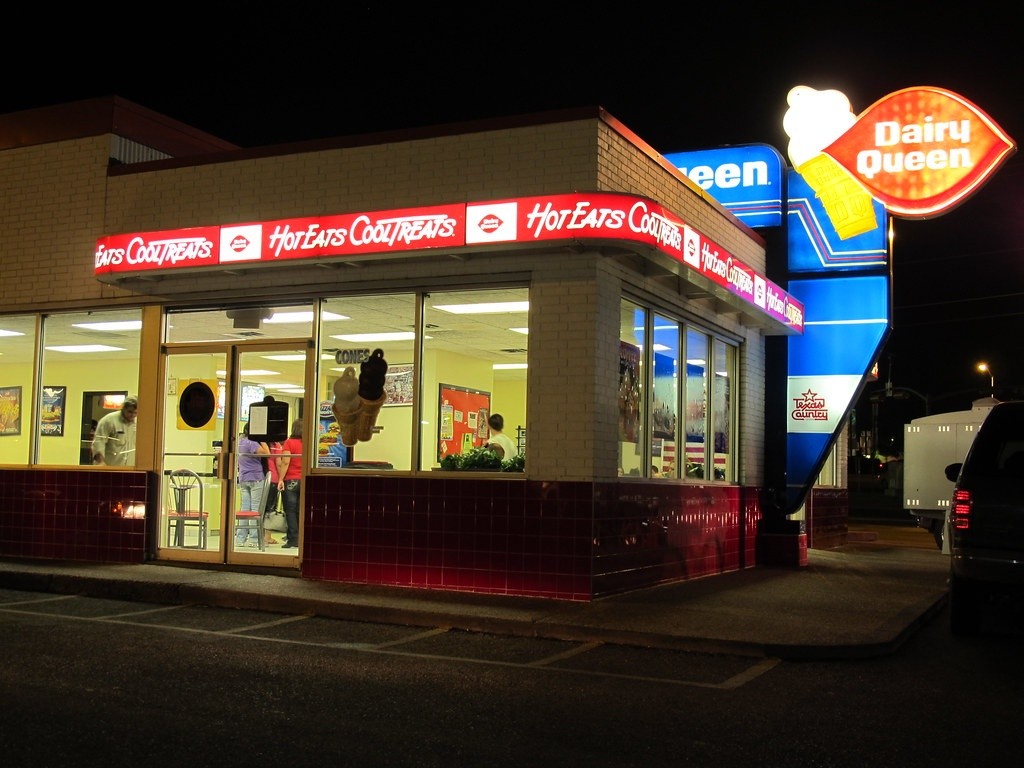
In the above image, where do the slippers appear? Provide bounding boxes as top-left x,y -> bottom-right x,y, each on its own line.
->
264,539 -> 278,544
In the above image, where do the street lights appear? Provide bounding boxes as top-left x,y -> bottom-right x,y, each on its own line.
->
977,362 -> 997,402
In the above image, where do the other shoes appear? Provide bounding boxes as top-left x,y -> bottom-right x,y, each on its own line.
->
236,542 -> 244,546
281,543 -> 298,548
248,542 -> 257,547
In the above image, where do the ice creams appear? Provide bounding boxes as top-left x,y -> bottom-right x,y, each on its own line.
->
332,347 -> 389,445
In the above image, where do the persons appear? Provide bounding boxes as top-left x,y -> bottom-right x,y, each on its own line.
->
277,418 -> 305,548
486,442 -> 506,461
234,422 -> 271,548
91,394 -> 139,466
483,412 -> 521,463
262,441 -> 284,545
616,457 -> 728,483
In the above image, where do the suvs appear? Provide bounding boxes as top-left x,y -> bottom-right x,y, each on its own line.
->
941,402 -> 1023,655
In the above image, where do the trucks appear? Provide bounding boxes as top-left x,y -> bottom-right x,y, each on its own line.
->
901,394 -> 1005,552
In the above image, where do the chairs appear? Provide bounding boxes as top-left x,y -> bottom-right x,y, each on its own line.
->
236,471 -> 272,552
165,469 -> 209,550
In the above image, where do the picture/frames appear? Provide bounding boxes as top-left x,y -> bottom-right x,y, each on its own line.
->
40,386 -> 66,437
382,362 -> 415,406
0,386 -> 22,435
